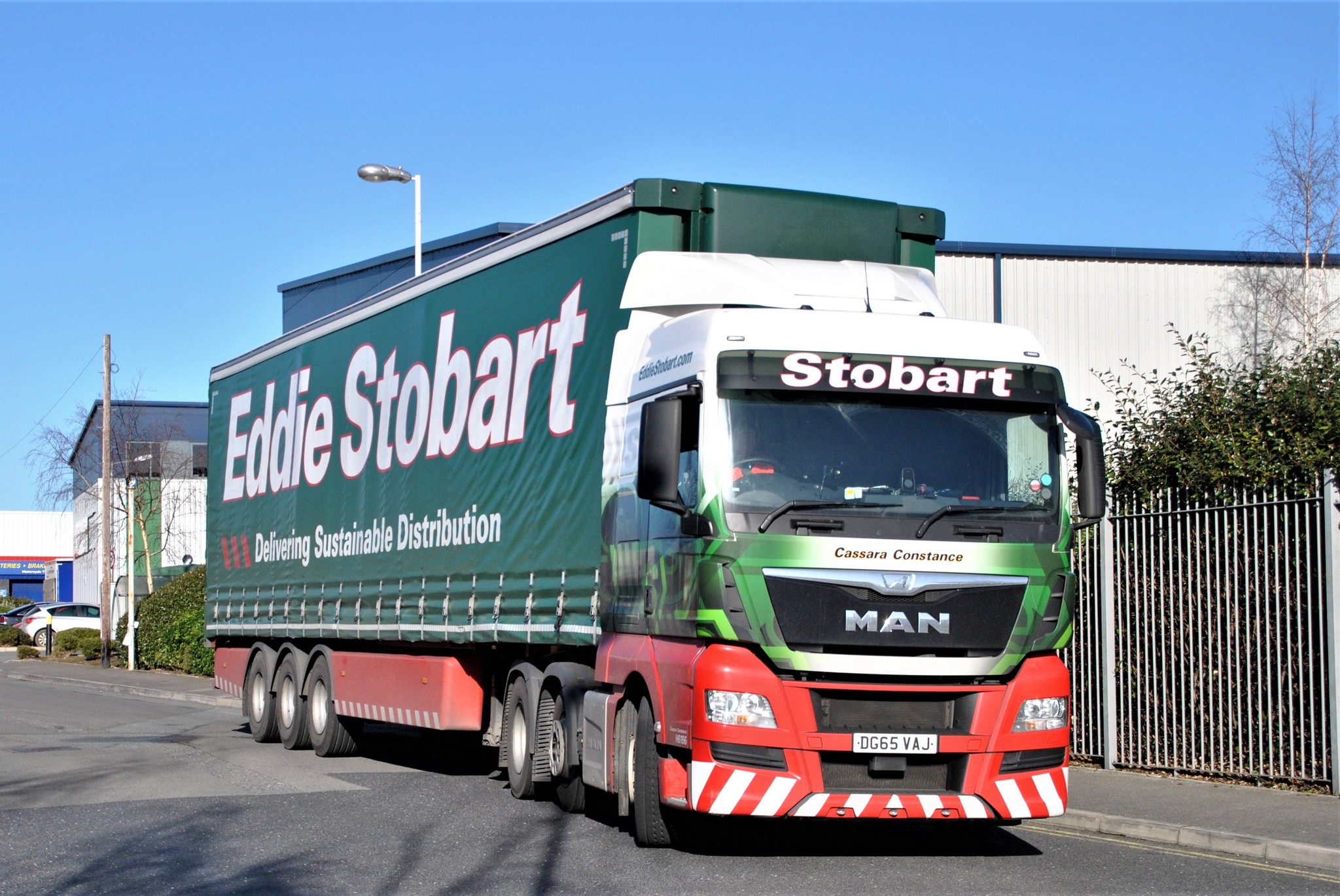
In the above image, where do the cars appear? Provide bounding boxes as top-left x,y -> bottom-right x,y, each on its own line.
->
0,601 -> 61,630
22,602 -> 101,648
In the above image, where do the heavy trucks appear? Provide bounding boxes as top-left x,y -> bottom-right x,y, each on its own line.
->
205,177 -> 1109,851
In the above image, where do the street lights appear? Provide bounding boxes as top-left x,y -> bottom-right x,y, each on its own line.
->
124,471 -> 138,670
357,162 -> 422,279
109,454 -> 153,571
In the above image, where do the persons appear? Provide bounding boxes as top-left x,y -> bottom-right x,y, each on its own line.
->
733,416 -> 784,483
